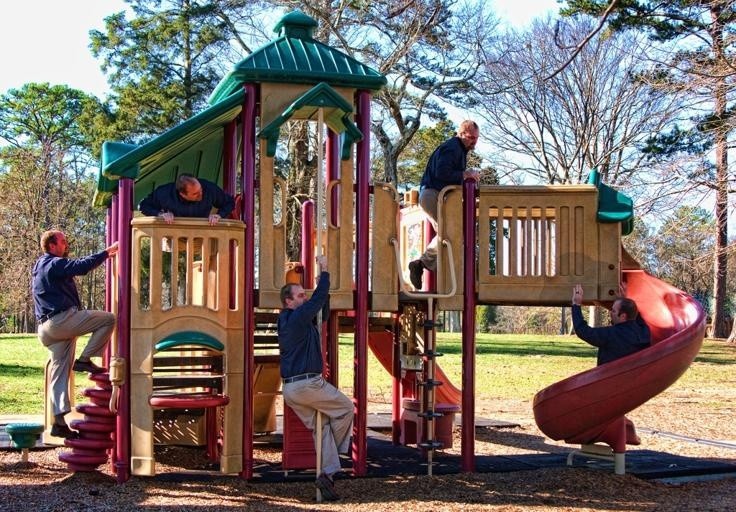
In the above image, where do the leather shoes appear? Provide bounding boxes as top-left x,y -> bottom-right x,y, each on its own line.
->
51,424 -> 79,438
72,358 -> 107,373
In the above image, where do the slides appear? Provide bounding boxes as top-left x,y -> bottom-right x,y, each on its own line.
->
533,243 -> 707,443
367,328 -> 461,411
246,365 -> 281,436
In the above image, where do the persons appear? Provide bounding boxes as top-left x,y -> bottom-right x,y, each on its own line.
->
408,119 -> 480,289
137,173 -> 235,312
276,255 -> 357,504
570,283 -> 652,372
30,229 -> 118,439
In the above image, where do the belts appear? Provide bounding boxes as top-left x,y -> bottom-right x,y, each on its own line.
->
421,185 -> 440,190
37,308 -> 66,325
283,375 -> 316,384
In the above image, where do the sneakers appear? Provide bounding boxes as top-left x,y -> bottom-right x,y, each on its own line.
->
409,260 -> 424,289
314,472 -> 341,500
